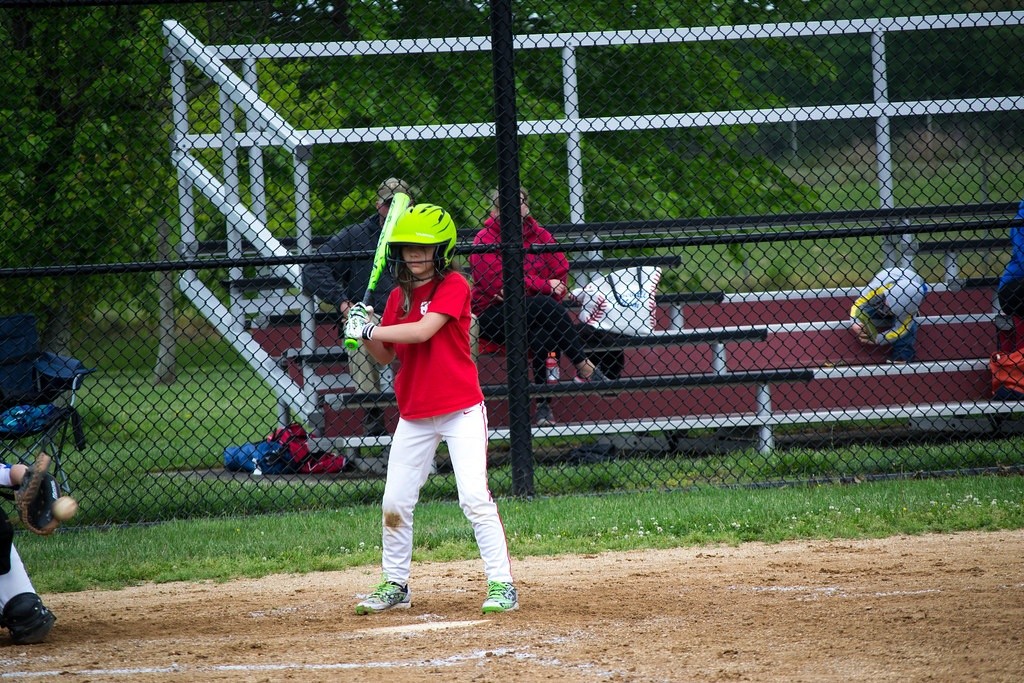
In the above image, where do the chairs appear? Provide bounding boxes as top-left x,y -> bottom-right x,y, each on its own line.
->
0,315 -> 96,494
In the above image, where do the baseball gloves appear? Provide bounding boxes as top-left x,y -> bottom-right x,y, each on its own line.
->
15,451 -> 59,535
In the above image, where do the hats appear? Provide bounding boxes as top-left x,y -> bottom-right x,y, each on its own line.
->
379,177 -> 410,204
888,281 -> 924,317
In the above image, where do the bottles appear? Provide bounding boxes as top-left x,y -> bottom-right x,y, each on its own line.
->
546,352 -> 559,384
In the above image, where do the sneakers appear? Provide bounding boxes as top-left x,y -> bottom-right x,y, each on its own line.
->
535,403 -> 555,426
357,572 -> 410,614
482,580 -> 519,612
574,367 -> 619,399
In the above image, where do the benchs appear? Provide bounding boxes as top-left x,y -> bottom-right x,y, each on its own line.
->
244,282 -> 1024,455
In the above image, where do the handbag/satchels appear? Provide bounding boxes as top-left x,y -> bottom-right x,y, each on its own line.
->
991,348 -> 1024,402
579,265 -> 661,336
226,421 -> 346,475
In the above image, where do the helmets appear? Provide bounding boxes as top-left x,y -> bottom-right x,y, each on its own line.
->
384,203 -> 456,282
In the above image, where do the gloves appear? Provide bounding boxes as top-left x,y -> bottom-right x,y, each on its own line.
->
348,302 -> 374,323
343,317 -> 376,341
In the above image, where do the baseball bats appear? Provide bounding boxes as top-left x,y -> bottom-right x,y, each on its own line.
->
344,192 -> 410,350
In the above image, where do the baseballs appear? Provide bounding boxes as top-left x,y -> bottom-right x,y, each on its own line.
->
53,495 -> 78,520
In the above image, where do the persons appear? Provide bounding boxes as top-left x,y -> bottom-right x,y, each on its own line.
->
0,462 -> 55,645
342,202 -> 519,612
998,199 -> 1024,317
469,186 -> 618,427
301,178 -> 414,435
